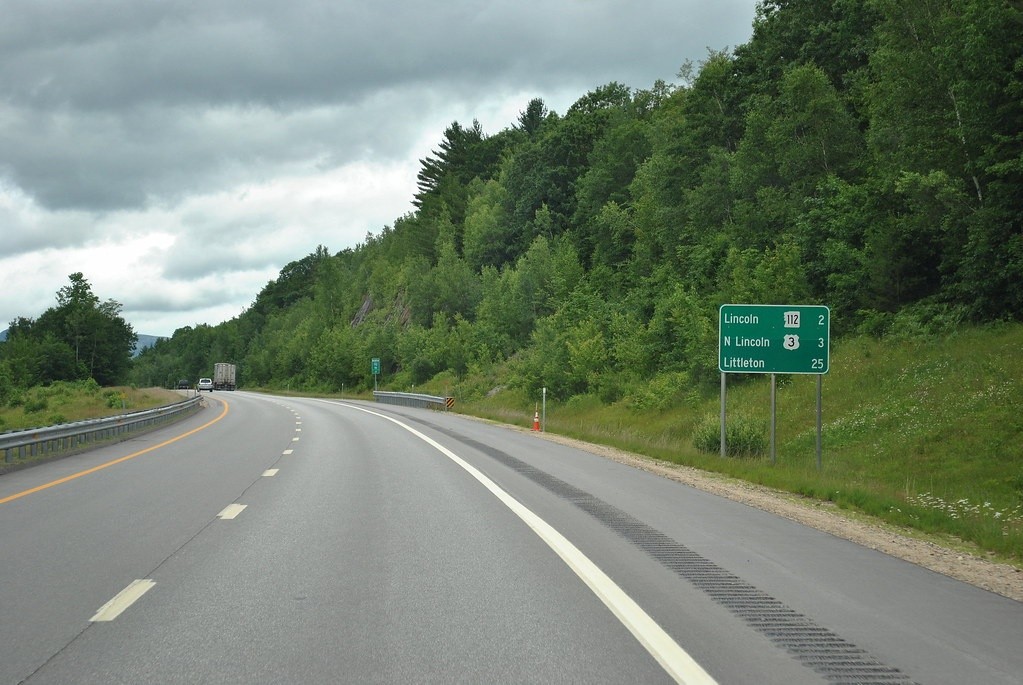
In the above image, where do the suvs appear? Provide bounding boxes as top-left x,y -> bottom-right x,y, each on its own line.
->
196,378 -> 213,392
178,380 -> 189,389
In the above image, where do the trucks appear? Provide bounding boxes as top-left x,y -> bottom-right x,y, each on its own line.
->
212,362 -> 236,391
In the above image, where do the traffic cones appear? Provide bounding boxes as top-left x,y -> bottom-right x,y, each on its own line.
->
530,411 -> 541,431
